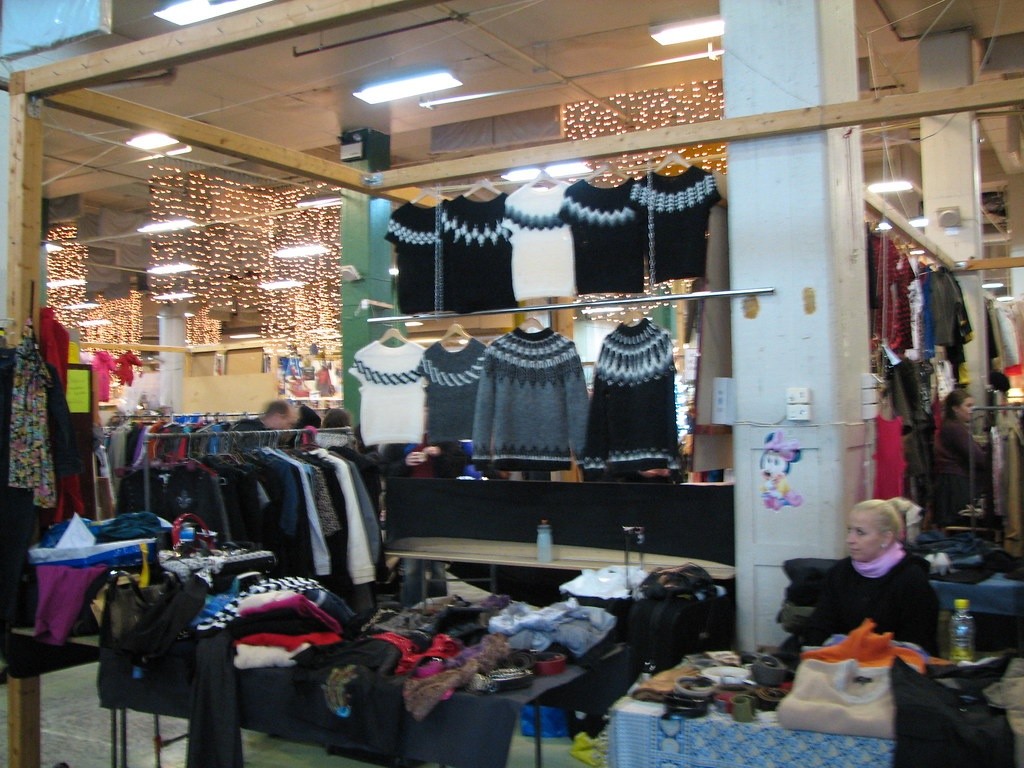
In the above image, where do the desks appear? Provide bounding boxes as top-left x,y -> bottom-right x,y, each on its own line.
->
13,615 -> 622,768
930,570 -> 1023,658
605,655 -> 1024,767
382,533 -> 737,615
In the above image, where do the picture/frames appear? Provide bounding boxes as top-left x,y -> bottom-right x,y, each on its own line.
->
213,353 -> 227,376
262,353 -> 273,373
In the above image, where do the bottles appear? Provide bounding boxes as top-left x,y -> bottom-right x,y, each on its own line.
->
950,599 -> 974,662
537,520 -> 552,563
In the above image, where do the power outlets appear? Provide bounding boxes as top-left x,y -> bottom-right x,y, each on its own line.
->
787,387 -> 810,404
787,405 -> 811,421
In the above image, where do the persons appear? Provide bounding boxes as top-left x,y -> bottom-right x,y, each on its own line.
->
607,455 -> 688,486
937,390 -> 990,535
385,393 -> 470,480
779,496 -> 941,659
232,402 -> 292,431
321,408 -> 352,428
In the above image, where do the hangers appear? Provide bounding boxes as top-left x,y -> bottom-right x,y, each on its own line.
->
411,148 -> 691,207
106,409 -> 358,465
373,306 -> 645,347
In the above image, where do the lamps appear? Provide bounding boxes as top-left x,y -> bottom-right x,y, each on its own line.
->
649,12 -> 725,46
353,66 -> 464,104
152,0 -> 278,26
